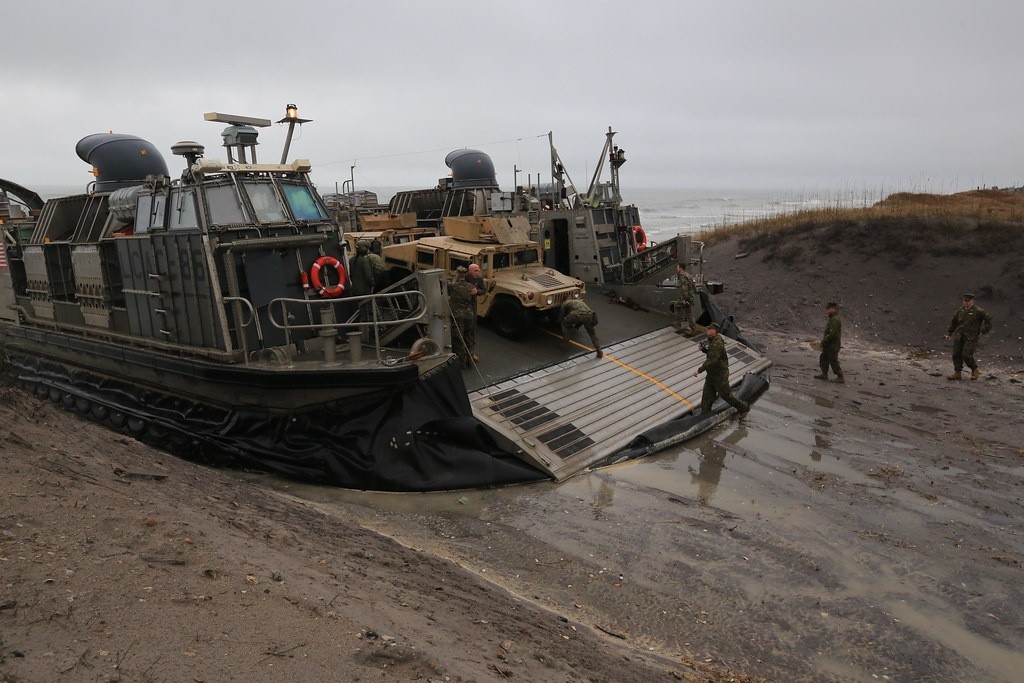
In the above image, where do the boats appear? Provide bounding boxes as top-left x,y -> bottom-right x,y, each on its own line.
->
0,104 -> 768,484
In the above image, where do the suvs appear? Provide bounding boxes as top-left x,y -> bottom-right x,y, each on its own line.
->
342,213 -> 589,338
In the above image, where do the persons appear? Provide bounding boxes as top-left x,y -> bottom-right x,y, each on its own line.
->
814,302 -> 845,383
349,240 -> 390,330
671,263 -> 696,336
694,322 -> 751,421
945,294 -> 992,380
557,295 -> 603,357
446,263 -> 487,363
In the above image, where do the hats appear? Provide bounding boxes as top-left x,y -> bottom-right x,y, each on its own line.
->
963,294 -> 975,302
705,322 -> 720,331
455,266 -> 468,276
825,301 -> 837,307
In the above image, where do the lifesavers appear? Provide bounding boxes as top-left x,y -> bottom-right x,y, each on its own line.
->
633,225 -> 648,250
310,255 -> 347,296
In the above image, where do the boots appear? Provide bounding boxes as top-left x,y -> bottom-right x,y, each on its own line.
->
970,367 -> 979,379
830,374 -> 844,383
947,370 -> 961,380
814,371 -> 828,380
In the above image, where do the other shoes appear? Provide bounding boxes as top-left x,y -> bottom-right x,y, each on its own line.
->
470,351 -> 479,363
697,413 -> 704,416
735,401 -> 750,420
596,348 -> 603,359
673,319 -> 681,328
687,327 -> 696,336
559,336 -> 569,346
458,354 -> 467,369
